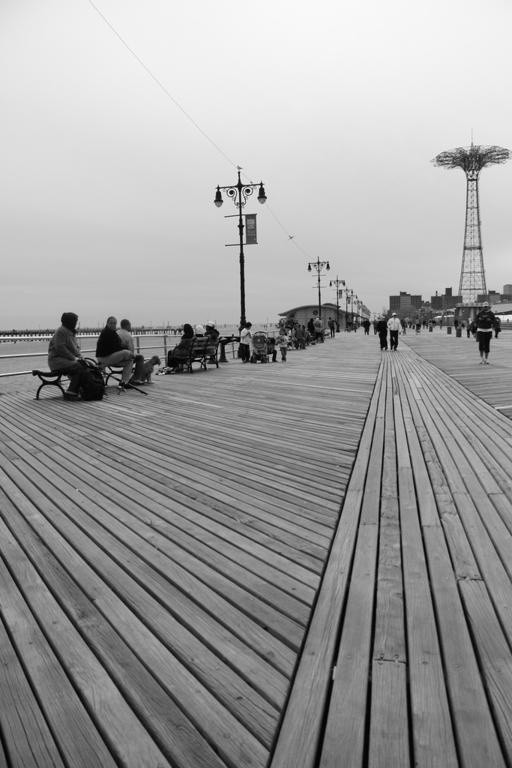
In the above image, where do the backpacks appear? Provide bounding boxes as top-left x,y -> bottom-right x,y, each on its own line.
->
77,366 -> 104,400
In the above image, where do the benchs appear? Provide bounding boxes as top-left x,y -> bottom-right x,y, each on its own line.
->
33,326 -> 332,401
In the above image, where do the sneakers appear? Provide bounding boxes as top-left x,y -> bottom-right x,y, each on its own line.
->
65,392 -> 79,400
479,360 -> 490,365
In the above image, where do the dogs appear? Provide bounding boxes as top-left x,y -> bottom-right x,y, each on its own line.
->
139,356 -> 161,384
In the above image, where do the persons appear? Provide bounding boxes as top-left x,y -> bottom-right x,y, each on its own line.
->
95,316 -> 135,390
47,312 -> 86,399
116,318 -> 144,384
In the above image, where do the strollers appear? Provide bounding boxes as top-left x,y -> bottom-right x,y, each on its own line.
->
250,330 -> 269,364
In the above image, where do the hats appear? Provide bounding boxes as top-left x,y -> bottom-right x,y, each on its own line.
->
482,301 -> 488,306
62,312 -> 78,327
205,321 -> 215,328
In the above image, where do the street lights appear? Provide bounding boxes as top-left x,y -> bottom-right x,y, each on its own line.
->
213,164 -> 267,336
329,274 -> 346,332
307,255 -> 330,321
338,286 -> 372,332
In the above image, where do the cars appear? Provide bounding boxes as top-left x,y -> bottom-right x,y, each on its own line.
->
494,310 -> 512,325
